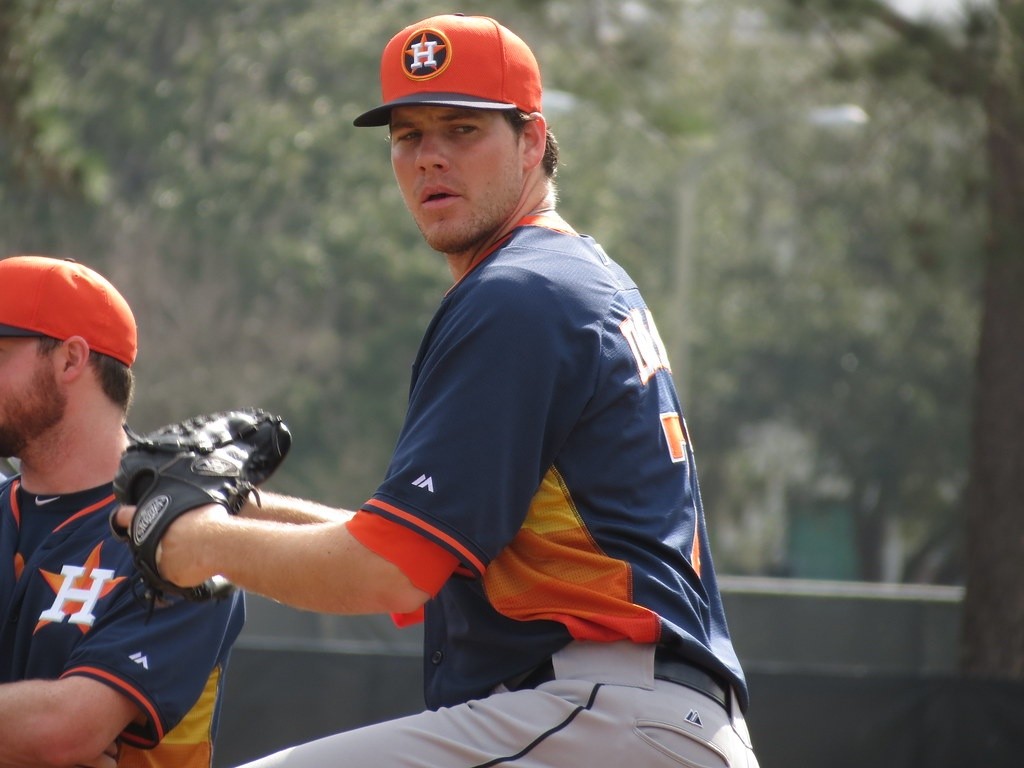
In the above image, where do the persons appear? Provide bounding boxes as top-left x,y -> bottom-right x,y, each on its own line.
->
107,13 -> 760,768
0,257 -> 247,767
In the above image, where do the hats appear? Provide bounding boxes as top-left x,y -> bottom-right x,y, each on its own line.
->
351,12 -> 543,128
0,254 -> 139,368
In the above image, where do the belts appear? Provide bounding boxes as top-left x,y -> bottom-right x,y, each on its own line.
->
504,656 -> 735,718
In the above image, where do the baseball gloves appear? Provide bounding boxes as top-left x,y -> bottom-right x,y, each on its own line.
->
110,406 -> 294,606
